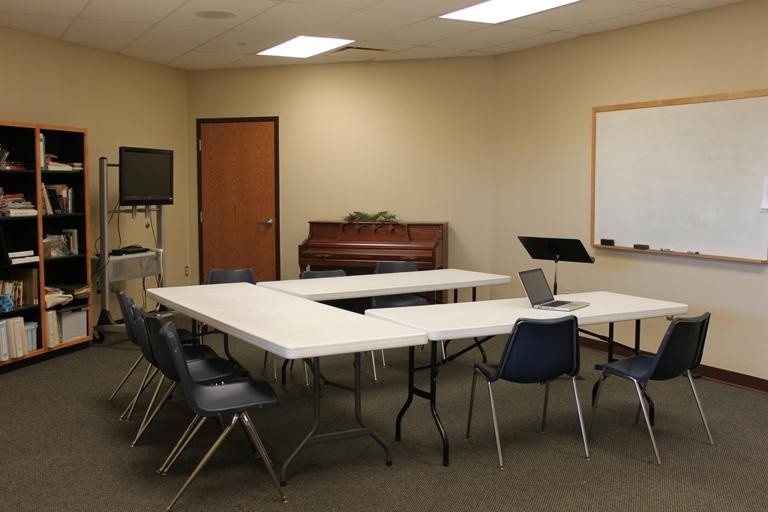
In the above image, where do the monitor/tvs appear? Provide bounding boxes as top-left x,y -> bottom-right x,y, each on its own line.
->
119,146 -> 173,206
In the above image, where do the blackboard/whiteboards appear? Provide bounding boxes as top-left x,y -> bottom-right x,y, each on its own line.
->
590,88 -> 768,265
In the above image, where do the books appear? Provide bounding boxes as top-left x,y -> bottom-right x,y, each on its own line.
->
0,133 -> 90,362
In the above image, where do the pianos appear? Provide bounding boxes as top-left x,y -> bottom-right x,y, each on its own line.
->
298,219 -> 447,307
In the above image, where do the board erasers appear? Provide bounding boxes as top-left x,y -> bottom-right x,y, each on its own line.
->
601,239 -> 614,245
634,245 -> 649,249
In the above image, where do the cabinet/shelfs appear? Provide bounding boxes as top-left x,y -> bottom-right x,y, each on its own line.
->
0,121 -> 95,372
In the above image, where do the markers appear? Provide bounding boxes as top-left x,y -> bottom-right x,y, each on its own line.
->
661,248 -> 672,251
687,251 -> 699,254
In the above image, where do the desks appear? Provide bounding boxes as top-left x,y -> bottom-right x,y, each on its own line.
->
256,269 -> 513,384
365,290 -> 691,466
142,282 -> 428,486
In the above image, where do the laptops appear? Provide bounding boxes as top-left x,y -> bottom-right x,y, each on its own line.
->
517,268 -> 590,312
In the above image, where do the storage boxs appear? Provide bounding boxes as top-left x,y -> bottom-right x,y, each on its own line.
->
58,310 -> 88,344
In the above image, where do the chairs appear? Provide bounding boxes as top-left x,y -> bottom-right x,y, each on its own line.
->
588,311 -> 715,466
156,320 -> 288,510
199,269 -> 277,381
109,290 -> 251,447
291,269 -> 379,386
464,314 -> 590,470
372,261 -> 446,367
